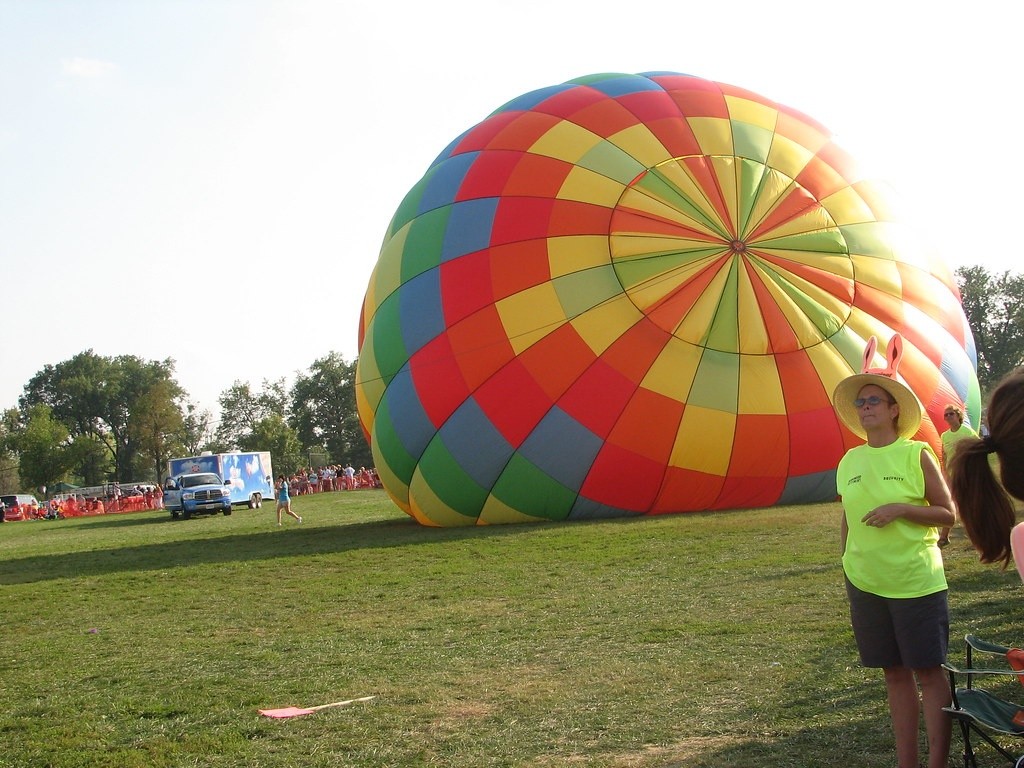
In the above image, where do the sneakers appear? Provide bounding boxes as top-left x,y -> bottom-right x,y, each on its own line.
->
297,517 -> 302,524
273,523 -> 282,527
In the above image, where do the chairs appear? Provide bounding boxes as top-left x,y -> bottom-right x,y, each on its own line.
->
939,633 -> 1024,768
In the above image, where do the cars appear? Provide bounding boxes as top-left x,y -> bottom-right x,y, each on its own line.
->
162,472 -> 232,521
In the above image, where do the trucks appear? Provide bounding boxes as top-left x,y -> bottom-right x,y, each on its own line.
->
166,449 -> 275,509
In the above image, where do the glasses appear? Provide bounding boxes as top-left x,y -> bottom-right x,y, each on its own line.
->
852,395 -> 893,407
943,412 -> 955,417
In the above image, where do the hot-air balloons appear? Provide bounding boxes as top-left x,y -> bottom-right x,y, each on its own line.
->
354,71 -> 982,528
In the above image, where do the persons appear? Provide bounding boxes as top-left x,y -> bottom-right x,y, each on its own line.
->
275,473 -> 302,526
937,404 -> 981,546
832,372 -> 959,768
134,484 -> 163,510
944,366 -> 1024,587
29,493 -> 99,520
288,464 -> 379,496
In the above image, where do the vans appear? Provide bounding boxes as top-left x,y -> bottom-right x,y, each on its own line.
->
0,494 -> 39,520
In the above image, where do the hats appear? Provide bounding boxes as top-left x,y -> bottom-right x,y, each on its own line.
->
51,504 -> 54,506
832,372 -> 922,443
278,475 -> 283,479
